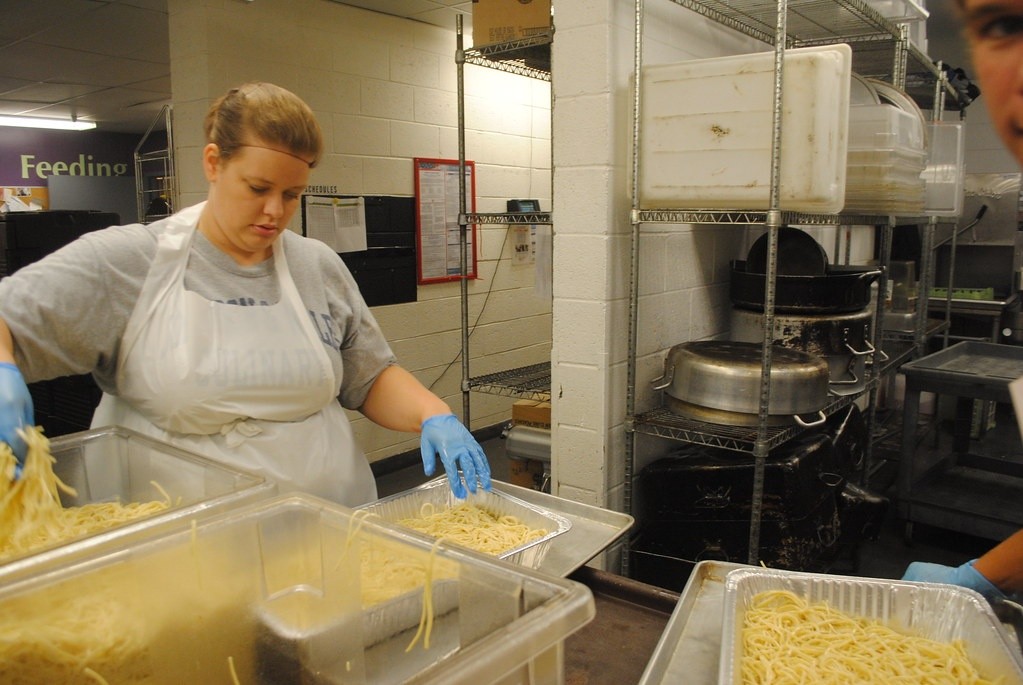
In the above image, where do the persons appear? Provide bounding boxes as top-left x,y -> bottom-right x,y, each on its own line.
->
0,82 -> 492,508
901,0 -> 1023,608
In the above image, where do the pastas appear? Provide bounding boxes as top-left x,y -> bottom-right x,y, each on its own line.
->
270,497 -> 549,652
0,421 -> 264,685
739,588 -> 1004,685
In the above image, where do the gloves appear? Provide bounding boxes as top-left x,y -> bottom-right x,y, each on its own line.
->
0,362 -> 35,480
420,414 -> 492,499
901,560 -> 1017,606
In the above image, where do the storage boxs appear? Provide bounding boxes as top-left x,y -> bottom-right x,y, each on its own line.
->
631,40 -> 963,217
510,394 -> 550,487
467,0 -> 553,49
0,420 -> 602,685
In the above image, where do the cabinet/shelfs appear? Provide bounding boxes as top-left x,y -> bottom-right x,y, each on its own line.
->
457,12 -> 550,492
135,102 -> 174,224
623,1 -> 966,569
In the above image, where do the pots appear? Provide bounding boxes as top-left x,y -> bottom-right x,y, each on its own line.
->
746,225 -> 828,275
729,304 -> 875,356
648,340 -> 829,429
669,397 -> 798,427
730,258 -> 883,314
818,354 -> 866,397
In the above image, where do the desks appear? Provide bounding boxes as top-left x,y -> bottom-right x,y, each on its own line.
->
898,342 -> 1022,547
